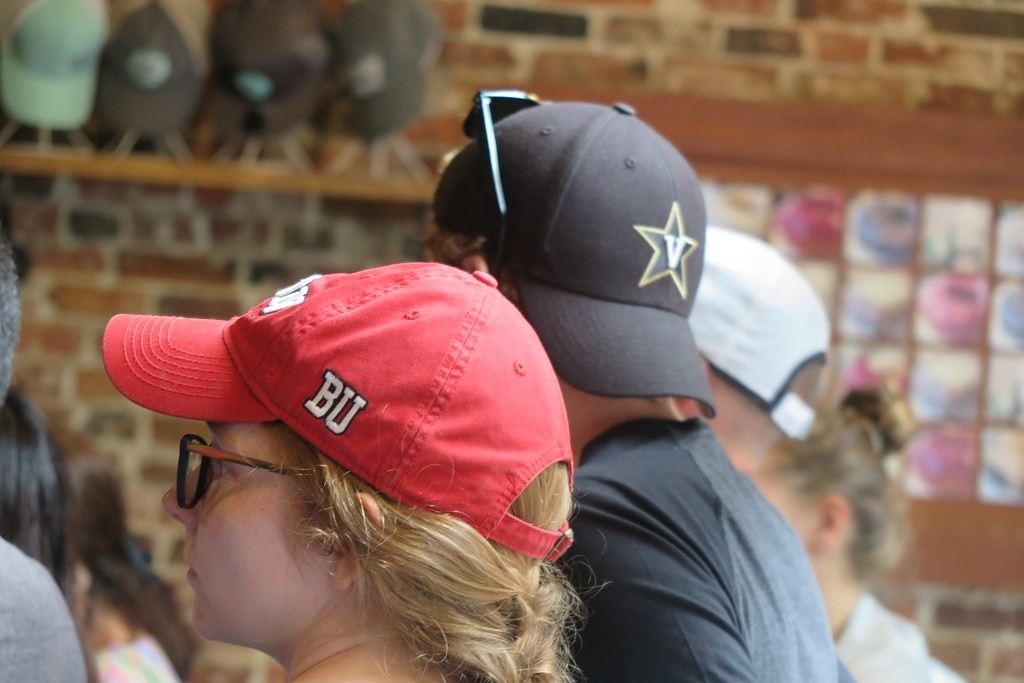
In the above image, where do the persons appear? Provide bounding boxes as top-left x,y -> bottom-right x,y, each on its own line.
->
422,88 -> 836,683
0,224 -> 200,683
674,228 -> 832,473
105,261 -> 575,683
757,382 -> 969,683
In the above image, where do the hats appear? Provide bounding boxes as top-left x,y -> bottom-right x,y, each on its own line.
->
688,226 -> 828,442
433,101 -> 718,418
101,261 -> 576,566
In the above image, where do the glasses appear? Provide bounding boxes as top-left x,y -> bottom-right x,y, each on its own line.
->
176,434 -> 304,509
463,91 -> 540,274
